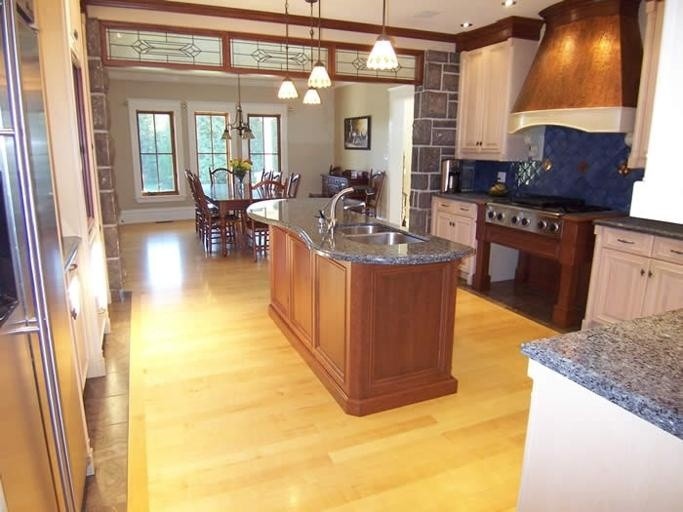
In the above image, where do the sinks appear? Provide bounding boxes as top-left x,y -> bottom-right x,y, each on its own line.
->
345,232 -> 431,246
335,222 -> 406,235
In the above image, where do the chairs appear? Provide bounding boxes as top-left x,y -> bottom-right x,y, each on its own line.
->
240,178 -> 288,262
358,168 -> 386,217
258,168 -> 271,190
208,166 -> 236,185
269,170 -> 281,190
183,167 -> 222,255
285,172 -> 301,200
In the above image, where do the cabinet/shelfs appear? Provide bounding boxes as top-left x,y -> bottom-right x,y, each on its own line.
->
319,173 -> 368,200
453,15 -> 545,163
626,0 -> 666,173
581,215 -> 683,333
429,192 -> 522,286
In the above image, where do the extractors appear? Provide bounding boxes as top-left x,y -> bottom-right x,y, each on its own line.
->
507,1 -> 641,135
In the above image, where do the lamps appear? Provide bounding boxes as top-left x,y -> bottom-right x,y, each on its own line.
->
306,1 -> 333,90
277,1 -> 298,103
300,1 -> 323,105
217,73 -> 255,142
366,0 -> 400,72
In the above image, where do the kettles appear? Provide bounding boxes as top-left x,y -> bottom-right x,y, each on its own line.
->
440,159 -> 463,195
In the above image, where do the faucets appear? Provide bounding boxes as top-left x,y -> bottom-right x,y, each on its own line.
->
318,185 -> 375,240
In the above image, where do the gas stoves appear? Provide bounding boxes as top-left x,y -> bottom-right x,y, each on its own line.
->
484,190 -> 611,240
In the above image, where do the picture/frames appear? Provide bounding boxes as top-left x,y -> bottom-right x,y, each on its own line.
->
343,115 -> 371,150
